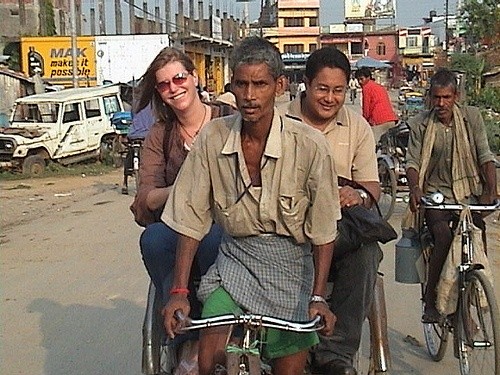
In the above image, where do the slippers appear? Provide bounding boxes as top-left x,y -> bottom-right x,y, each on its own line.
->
175,360 -> 199,375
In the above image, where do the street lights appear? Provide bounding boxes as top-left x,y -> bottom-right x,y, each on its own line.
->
430,0 -> 449,62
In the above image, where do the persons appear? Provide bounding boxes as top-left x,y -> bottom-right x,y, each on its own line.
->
130,46 -> 239,375
348,75 -> 359,98
276,47 -> 381,375
355,68 -> 398,146
404,70 -> 500,335
201,83 -> 238,111
406,65 -> 415,82
160,37 -> 342,375
290,79 -> 306,101
121,95 -> 155,193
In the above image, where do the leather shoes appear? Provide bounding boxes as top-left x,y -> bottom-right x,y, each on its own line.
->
327,360 -> 357,375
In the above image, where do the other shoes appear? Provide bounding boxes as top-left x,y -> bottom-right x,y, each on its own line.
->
122,187 -> 128,194
423,304 -> 441,320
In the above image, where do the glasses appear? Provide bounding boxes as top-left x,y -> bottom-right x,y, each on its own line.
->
155,69 -> 192,93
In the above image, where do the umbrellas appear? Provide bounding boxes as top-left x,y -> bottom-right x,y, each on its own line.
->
350,56 -> 393,68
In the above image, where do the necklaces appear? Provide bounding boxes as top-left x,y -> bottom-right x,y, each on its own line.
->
179,104 -> 206,147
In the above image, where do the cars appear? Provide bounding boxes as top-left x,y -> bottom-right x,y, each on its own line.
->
396,79 -> 428,116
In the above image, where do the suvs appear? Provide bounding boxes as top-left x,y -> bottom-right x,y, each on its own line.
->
0,82 -> 127,180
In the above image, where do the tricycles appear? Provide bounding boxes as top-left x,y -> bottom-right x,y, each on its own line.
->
376,118 -> 410,221
141,268 -> 391,375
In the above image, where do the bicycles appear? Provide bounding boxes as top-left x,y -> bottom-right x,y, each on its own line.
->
394,192 -> 500,375
123,135 -> 146,187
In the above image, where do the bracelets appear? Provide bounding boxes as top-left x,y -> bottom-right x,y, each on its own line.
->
411,185 -> 418,190
356,190 -> 367,206
170,289 -> 190,294
309,296 -> 329,307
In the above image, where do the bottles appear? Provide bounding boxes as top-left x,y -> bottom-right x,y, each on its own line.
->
394,228 -> 423,283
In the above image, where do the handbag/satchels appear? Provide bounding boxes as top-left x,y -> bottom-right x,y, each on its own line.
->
336,175 -> 398,249
466,206 -> 494,306
435,206 -> 469,315
